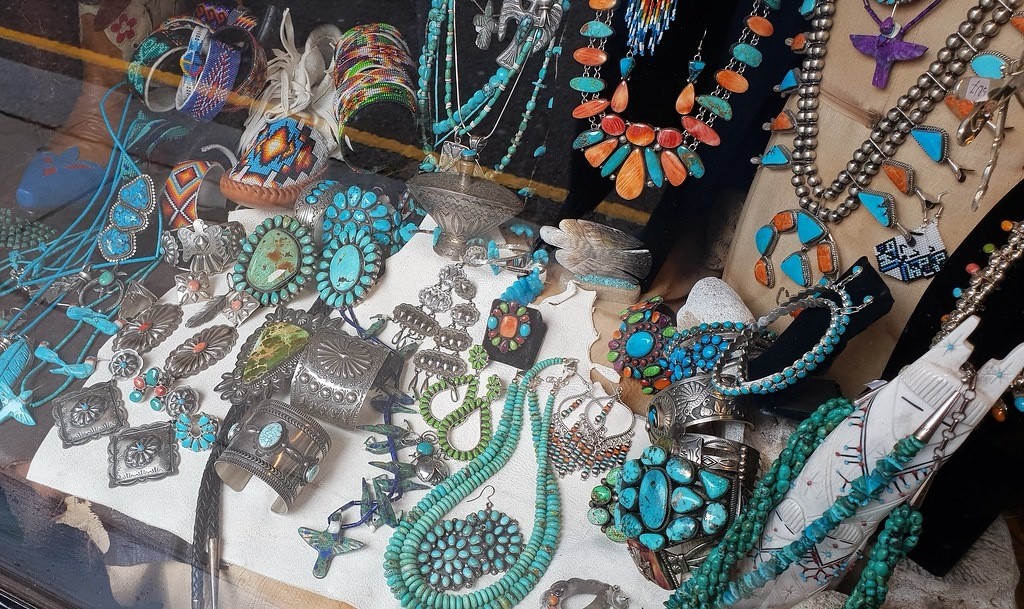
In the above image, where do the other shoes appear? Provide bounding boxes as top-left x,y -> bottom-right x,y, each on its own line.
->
219,8 -> 343,209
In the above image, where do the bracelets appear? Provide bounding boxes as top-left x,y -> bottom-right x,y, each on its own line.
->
713,282 -> 854,397
645,321 -> 777,476
213,399 -> 333,513
161,161 -> 228,239
126,4 -> 267,121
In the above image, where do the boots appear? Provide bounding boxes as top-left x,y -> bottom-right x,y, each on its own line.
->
16,0 -> 178,208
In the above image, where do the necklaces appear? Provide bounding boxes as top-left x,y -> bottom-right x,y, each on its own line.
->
662,362 -> 975,609
754,0 -> 1024,315
382,356 -> 579,609
569,0 -> 778,199
418,0 -> 571,184
